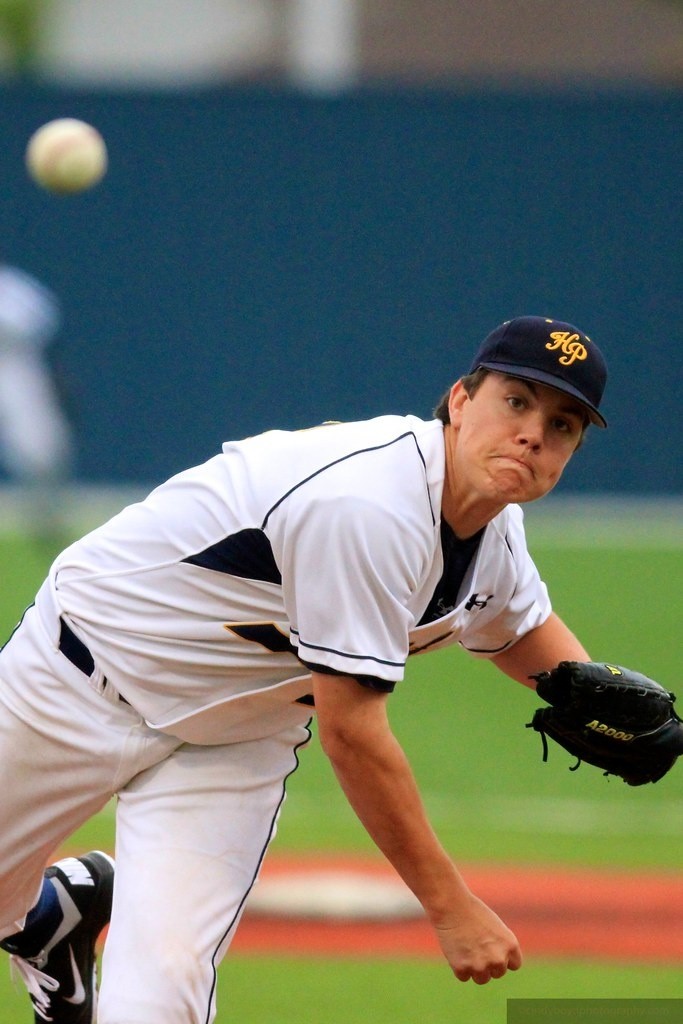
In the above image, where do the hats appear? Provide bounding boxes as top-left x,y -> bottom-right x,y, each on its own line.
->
469,315 -> 609,430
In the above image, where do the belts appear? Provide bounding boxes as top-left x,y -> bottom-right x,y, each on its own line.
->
56,616 -> 130,705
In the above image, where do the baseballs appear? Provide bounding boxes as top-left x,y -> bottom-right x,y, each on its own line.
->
25,116 -> 109,194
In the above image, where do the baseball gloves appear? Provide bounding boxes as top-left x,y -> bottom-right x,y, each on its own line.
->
523,658 -> 683,788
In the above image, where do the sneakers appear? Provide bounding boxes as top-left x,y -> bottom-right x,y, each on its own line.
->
0,849 -> 116,1024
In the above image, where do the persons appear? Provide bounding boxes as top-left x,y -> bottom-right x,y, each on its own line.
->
0,316 -> 609,1024
